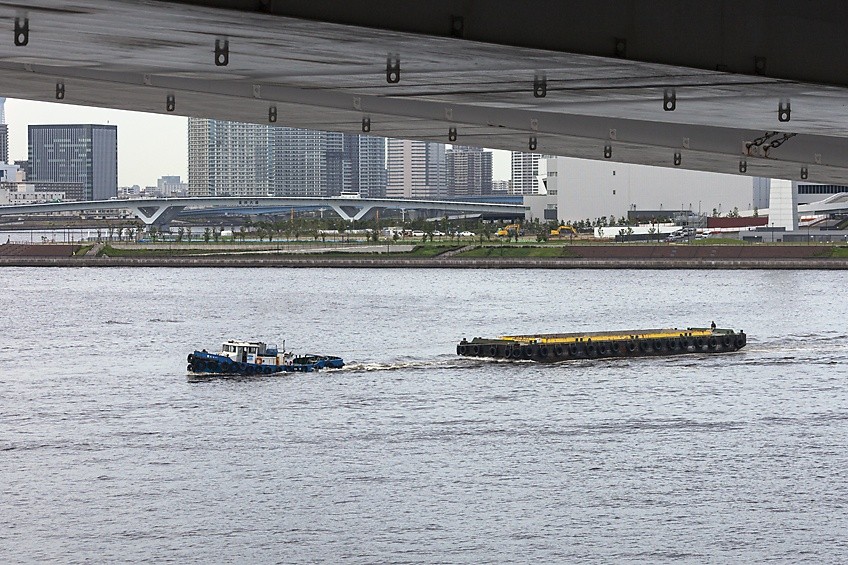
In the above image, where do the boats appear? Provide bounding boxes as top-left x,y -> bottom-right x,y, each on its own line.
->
187,339 -> 344,379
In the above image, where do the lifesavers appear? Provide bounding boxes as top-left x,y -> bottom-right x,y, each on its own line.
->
186,354 -> 239,372
325,362 -> 334,368
292,366 -> 308,372
459,336 -> 744,358
245,365 -> 288,374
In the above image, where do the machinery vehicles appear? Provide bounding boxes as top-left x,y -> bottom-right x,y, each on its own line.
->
548,226 -> 578,238
494,224 -> 520,236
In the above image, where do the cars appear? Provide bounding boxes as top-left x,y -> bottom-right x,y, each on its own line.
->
411,230 -> 475,236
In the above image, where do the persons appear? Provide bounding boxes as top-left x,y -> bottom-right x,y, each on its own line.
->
711,321 -> 716,329
73,251 -> 76,256
